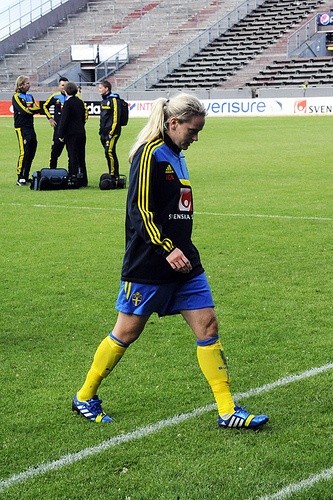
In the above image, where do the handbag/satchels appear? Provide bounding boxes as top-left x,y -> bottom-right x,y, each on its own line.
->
30,167 -> 77,190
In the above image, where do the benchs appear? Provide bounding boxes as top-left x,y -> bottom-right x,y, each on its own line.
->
0,0 -> 333,98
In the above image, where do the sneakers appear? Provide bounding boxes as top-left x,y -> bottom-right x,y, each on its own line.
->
16,178 -> 28,188
218,405 -> 269,428
71,394 -> 113,424
25,178 -> 32,184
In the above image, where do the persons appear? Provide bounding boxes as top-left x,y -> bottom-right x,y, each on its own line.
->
12,75 -> 41,186
99,81 -> 121,181
57,83 -> 87,187
43,78 -> 88,173
77,87 -> 82,94
72,93 -> 268,429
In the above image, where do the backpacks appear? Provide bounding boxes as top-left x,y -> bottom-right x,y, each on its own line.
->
118,97 -> 129,127
